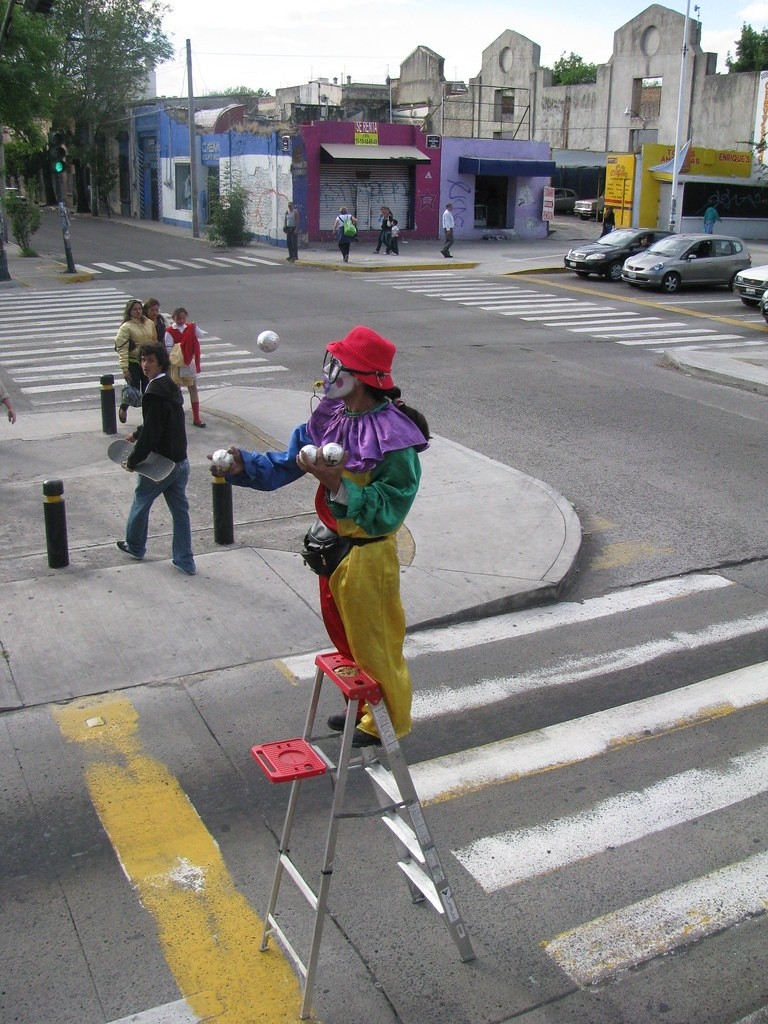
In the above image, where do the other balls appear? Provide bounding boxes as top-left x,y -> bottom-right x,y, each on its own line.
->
322,442 -> 344,466
212,448 -> 234,472
257,329 -> 280,353
299,444 -> 319,465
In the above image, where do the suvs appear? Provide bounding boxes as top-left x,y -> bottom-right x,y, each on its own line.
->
573,191 -> 604,222
554,188 -> 578,215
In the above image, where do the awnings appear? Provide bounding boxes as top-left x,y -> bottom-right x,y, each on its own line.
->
320,143 -> 430,161
457,157 -> 555,176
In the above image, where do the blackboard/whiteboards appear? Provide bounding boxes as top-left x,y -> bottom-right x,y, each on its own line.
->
681,180 -> 768,221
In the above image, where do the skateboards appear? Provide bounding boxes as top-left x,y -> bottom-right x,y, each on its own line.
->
106,438 -> 177,484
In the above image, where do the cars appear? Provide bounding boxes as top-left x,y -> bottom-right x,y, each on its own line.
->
6,188 -> 27,208
564,227 -> 677,282
621,234 -> 752,293
734,265 -> 768,324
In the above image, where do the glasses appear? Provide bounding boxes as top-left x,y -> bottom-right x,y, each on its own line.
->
322,349 -> 384,384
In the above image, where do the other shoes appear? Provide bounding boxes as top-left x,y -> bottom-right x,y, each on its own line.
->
291,257 -> 298,260
444,255 -> 454,258
193,420 -> 206,428
346,726 -> 387,749
171,560 -> 190,575
373,251 -> 379,254
440,250 -> 448,258
392,252 -> 399,256
286,257 -> 292,260
383,252 -> 389,255
325,711 -> 361,734
114,540 -> 144,560
343,255 -> 348,262
118,405 -> 128,424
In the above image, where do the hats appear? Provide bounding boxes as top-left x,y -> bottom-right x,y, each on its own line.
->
288,201 -> 294,206
327,325 -> 396,393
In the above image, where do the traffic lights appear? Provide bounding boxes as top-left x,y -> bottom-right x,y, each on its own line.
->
48,132 -> 69,174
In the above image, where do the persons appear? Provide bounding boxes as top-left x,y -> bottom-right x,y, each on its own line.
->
440,204 -> 454,258
115,342 -> 196,575
333,207 -> 357,262
704,203 -> 722,234
115,300 -> 158,422
0,380 -> 16,424
206,325 -> 429,746
374,207 -> 400,255
284,202 -> 299,261
142,298 -> 171,348
164,307 -> 207,427
600,206 -> 615,236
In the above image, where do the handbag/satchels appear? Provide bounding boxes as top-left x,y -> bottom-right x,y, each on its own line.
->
343,219 -> 358,238
120,379 -> 143,408
114,337 -> 136,356
299,520 -> 354,577
703,224 -> 707,232
387,214 -> 392,227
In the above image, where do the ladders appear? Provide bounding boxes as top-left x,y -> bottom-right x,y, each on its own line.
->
250,652 -> 477,1019
